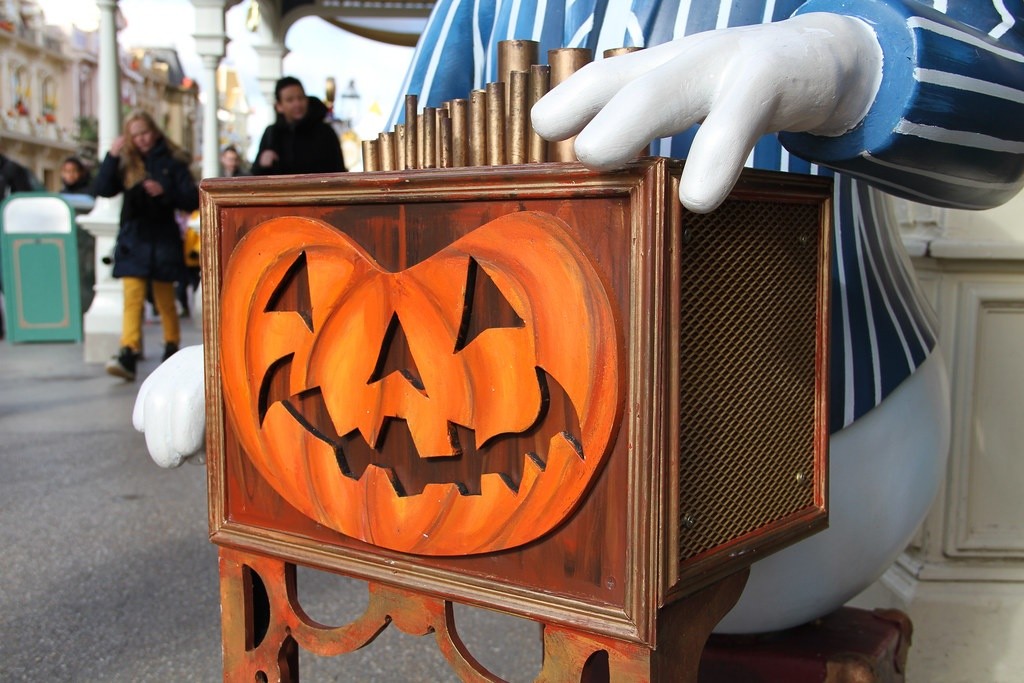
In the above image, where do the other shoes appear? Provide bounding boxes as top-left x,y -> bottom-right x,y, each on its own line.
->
105,346 -> 142,381
161,340 -> 180,362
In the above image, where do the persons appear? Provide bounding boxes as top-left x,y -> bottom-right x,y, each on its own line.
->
251,76 -> 349,176
59,157 -> 95,194
96,108 -> 250,380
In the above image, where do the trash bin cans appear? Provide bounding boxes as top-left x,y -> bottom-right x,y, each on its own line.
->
1,192 -> 95,347
